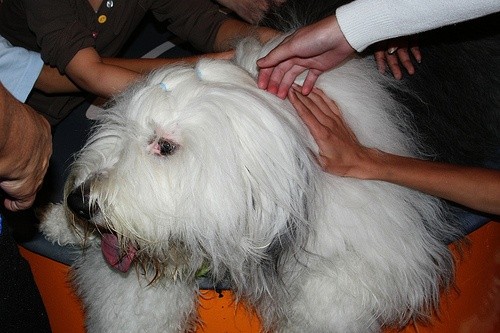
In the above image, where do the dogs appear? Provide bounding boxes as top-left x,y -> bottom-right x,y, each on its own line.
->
38,27 -> 471,333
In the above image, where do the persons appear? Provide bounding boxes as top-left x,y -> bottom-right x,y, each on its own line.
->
375,38 -> 423,82
0,33 -> 239,333
1,1 -> 281,257
287,84 -> 500,217
257,0 -> 500,99
